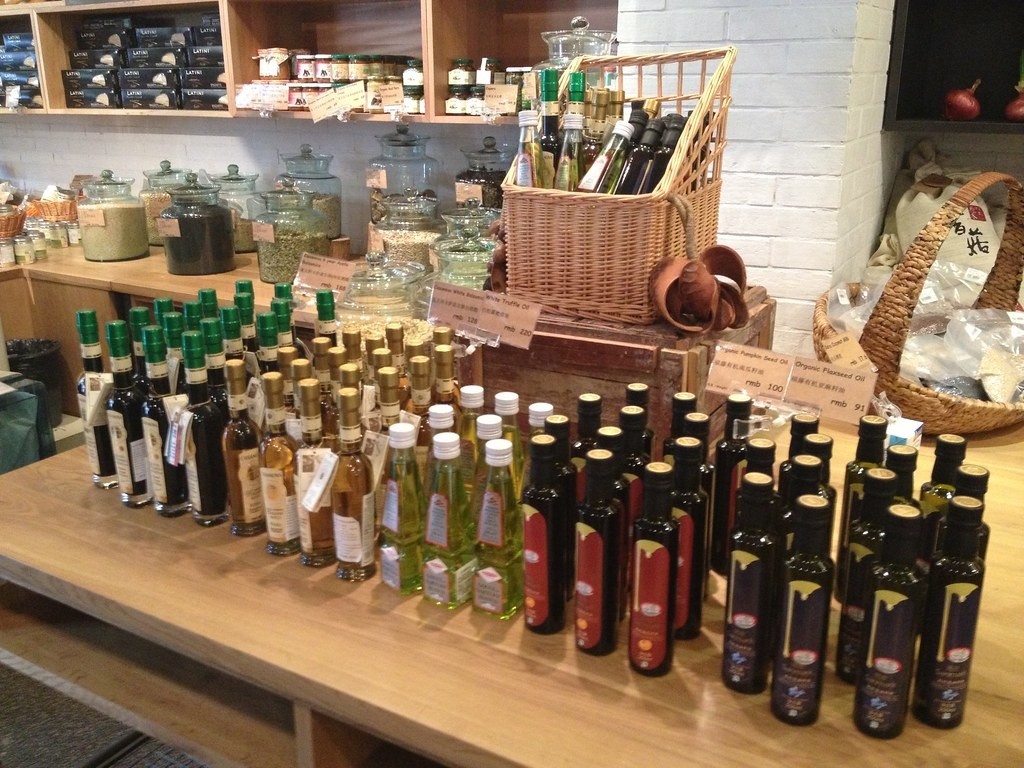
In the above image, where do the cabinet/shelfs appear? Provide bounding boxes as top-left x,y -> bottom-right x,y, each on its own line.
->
0,0 -> 618,124
881,0 -> 1024,134
0,244 -> 366,417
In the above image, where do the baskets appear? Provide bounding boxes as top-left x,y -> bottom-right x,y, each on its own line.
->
32,196 -> 84,222
811,170 -> 1023,437
0,208 -> 27,239
500,44 -> 737,329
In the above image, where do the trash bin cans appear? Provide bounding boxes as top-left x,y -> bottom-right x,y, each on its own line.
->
4,337 -> 64,428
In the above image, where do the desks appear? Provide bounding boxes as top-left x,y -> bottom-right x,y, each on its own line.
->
0,416 -> 1024,768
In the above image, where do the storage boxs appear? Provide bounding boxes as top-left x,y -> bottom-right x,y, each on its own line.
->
0,14 -> 229,111
455,283 -> 776,463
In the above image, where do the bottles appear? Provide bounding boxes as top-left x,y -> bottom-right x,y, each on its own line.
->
221,280 -> 375,584
526,414 -> 573,635
529,398 -> 553,425
712,391 -> 991,740
540,66 -> 560,192
575,382 -> 712,679
380,385 -> 525,619
516,110 -> 685,195
436,345 -> 461,404
641,100 -> 662,119
377,365 -> 403,424
586,87 -> 628,110
556,72 -> 584,191
373,346 -> 393,367
409,356 -> 436,416
76,289 -> 223,528
386,322 -> 410,367
404,326 -> 455,354
